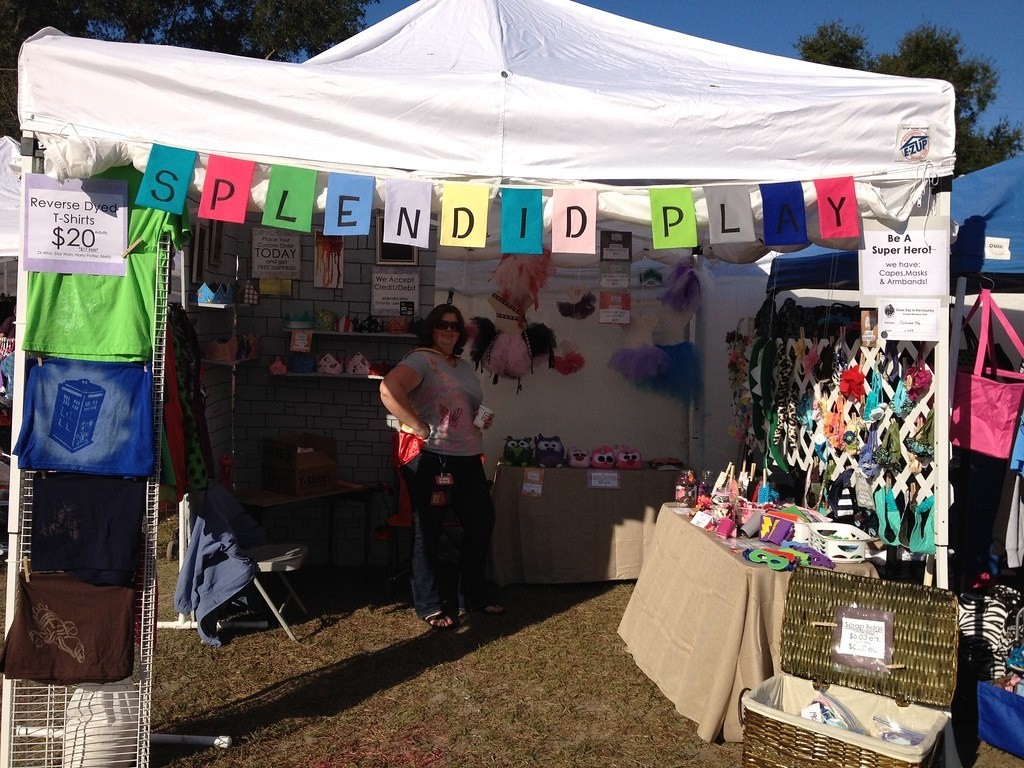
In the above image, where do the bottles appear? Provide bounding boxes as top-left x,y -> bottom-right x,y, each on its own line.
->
675,469 -> 697,507
697,470 -> 715,499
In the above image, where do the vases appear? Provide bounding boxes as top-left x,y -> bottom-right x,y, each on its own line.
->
698,468 -> 714,505
675,468 -> 697,508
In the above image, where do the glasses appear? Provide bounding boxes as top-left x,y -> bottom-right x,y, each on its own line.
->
434,319 -> 462,332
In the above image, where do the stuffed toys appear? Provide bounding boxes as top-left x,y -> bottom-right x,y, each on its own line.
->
503,433 -> 643,469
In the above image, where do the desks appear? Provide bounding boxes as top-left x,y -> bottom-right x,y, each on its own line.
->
231,480 -> 373,584
495,462 -> 691,589
618,501 -> 888,748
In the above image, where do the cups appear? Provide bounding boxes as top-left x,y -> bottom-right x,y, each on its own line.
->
473,404 -> 494,430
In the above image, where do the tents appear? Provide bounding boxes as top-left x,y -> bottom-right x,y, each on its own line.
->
0,135 -> 60,297
756,149 -> 1024,591
0,0 -> 957,768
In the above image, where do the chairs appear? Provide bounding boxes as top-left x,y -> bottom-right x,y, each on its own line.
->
374,432 -> 462,604
203,486 -> 309,646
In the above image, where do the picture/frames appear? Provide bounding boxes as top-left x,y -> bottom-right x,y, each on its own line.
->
192,223 -> 210,285
210,219 -> 226,267
375,213 -> 420,269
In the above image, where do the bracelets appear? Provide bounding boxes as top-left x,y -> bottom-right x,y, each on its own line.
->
421,420 -> 431,439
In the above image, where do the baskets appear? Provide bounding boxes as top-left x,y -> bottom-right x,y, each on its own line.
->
808,522 -> 871,565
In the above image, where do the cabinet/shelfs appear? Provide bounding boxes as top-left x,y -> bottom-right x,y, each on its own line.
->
189,301 -> 260,367
271,328 -> 419,381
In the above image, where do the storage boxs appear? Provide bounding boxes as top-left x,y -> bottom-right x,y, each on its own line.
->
737,505 -> 762,526
804,520 -> 873,565
262,427 -> 339,498
764,511 -> 834,543
976,677 -> 1024,761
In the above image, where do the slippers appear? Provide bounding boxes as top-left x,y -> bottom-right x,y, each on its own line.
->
422,611 -> 455,631
481,601 -> 505,615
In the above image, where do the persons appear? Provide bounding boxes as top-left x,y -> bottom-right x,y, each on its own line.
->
379,304 -> 504,627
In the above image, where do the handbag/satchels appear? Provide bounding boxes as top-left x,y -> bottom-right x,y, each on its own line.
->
948,288 -> 1024,458
